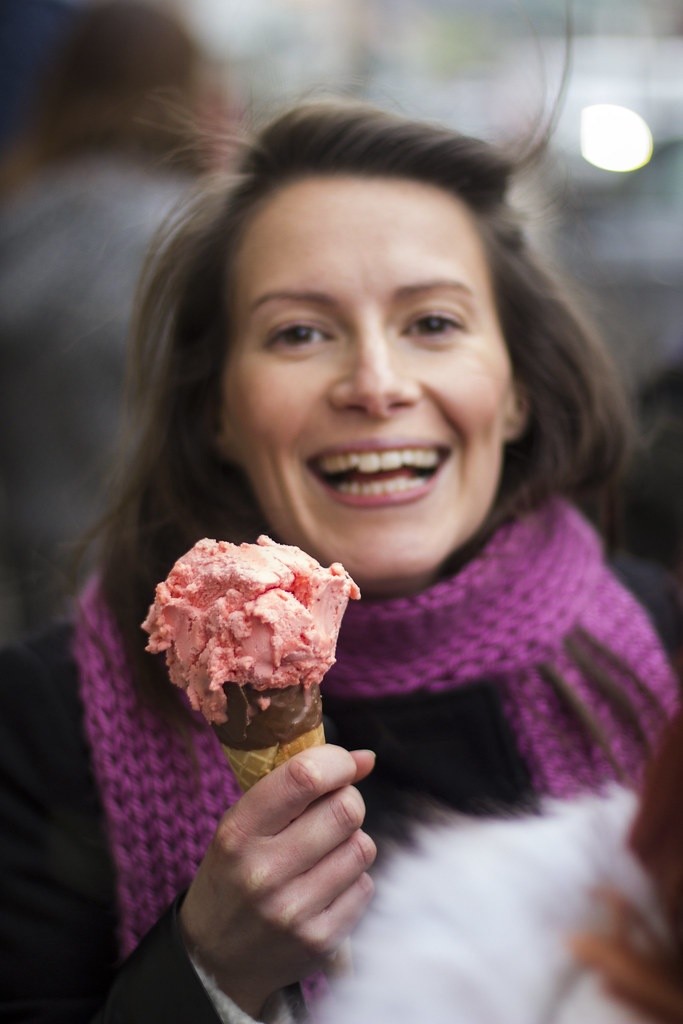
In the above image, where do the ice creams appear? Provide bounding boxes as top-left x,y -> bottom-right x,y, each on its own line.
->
140,534 -> 361,996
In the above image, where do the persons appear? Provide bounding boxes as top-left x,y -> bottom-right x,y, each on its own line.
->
0,1 -> 239,638
0,101 -> 683,1024
